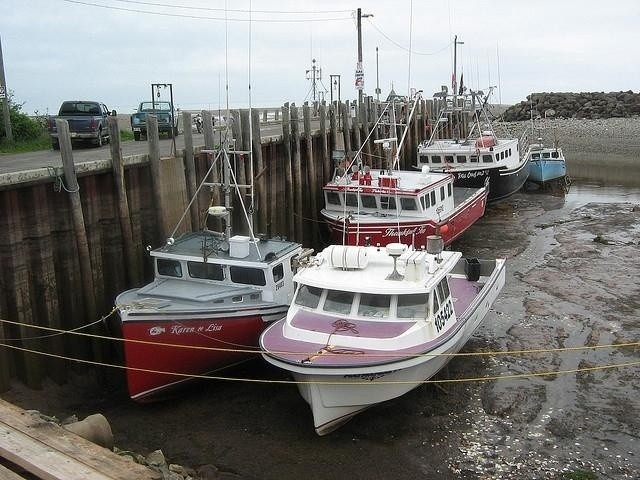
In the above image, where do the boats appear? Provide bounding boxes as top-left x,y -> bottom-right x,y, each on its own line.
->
113,113 -> 321,402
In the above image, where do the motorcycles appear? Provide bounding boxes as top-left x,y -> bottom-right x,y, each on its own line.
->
194,114 -> 204,133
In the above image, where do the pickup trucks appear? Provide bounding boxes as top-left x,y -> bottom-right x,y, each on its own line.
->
49,102 -> 116,148
131,101 -> 179,141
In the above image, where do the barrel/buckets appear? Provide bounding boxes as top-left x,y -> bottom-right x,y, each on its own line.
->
229,235 -> 250,258
326,244 -> 368,269
